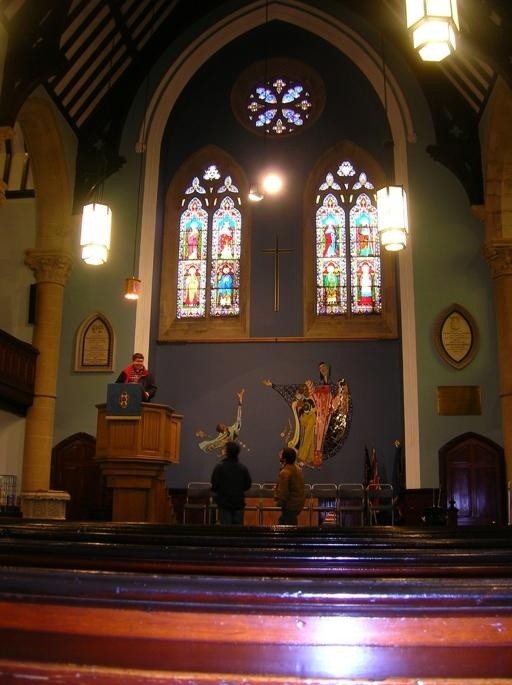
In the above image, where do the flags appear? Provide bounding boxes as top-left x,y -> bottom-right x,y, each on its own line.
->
363,446 -> 404,522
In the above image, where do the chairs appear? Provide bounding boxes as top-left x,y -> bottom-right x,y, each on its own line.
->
183,481 -> 400,528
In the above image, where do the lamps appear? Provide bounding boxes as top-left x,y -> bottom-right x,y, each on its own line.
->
406,0 -> 462,62
78,155 -> 112,266
376,58 -> 409,252
125,83 -> 141,301
249,182 -> 265,201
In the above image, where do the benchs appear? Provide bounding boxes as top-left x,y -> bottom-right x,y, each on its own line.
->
1,518 -> 512,685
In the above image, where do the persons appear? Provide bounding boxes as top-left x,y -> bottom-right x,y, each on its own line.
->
276,447 -> 306,525
114,353 -> 157,402
209,441 -> 252,526
